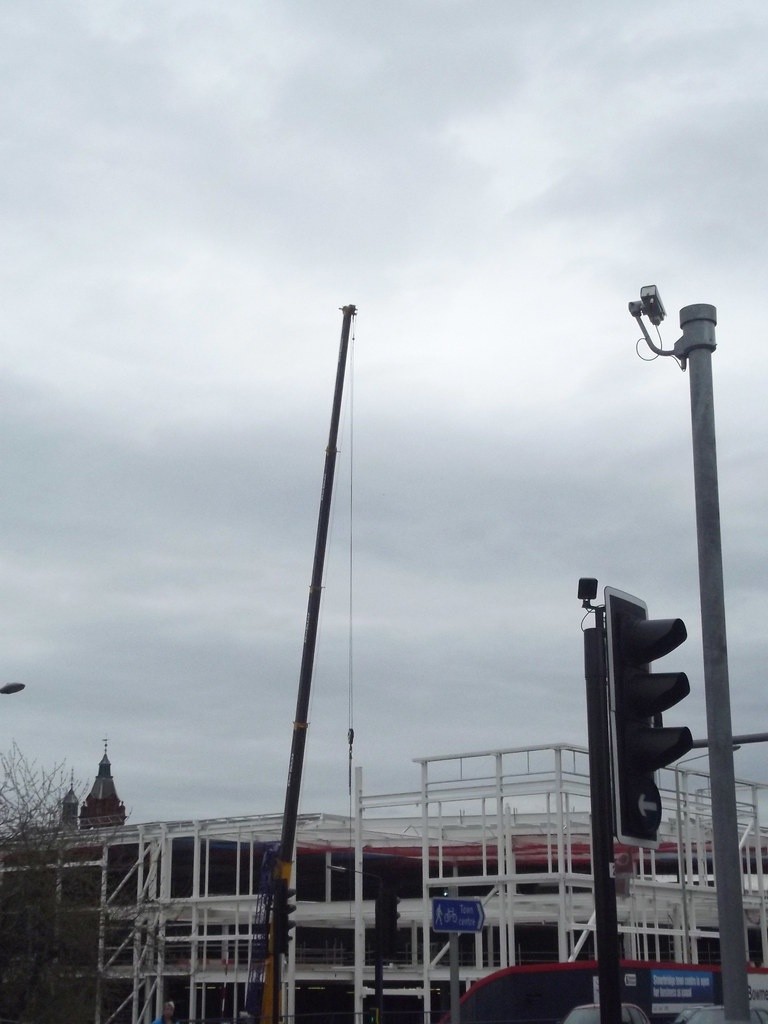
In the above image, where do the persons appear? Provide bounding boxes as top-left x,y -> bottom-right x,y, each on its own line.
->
151,1000 -> 180,1024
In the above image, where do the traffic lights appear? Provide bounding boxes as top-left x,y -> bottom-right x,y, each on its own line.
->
271,882 -> 298,957
600,586 -> 693,846
376,884 -> 403,939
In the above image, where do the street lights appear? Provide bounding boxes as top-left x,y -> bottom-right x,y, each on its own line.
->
630,282 -> 754,1024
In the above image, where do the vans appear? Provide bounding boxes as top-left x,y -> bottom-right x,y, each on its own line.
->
438,956 -> 767,1024
